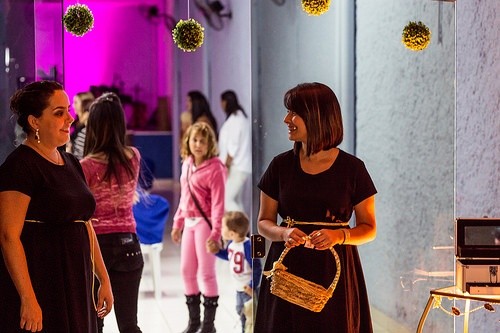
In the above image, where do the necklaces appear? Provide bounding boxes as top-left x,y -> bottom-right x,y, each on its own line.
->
25,136 -> 61,165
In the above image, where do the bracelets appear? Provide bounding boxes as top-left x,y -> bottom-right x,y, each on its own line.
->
339,228 -> 350,246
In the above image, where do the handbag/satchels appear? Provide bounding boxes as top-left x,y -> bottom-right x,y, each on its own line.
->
243,291 -> 258,333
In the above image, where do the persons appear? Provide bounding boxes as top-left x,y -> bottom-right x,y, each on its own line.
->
257,81 -> 377,333
0,82 -> 255,333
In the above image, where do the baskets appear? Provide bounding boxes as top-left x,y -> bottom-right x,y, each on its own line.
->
262,235 -> 342,313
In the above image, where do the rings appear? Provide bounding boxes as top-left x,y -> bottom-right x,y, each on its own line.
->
316,233 -> 321,236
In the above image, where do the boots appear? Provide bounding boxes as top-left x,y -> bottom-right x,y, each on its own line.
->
182,292 -> 201,333
194,294 -> 219,333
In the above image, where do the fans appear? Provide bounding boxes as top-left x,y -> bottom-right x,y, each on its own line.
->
138,5 -> 178,35
193,0 -> 232,32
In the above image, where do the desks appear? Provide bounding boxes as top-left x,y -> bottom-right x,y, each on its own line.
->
415,284 -> 500,333
